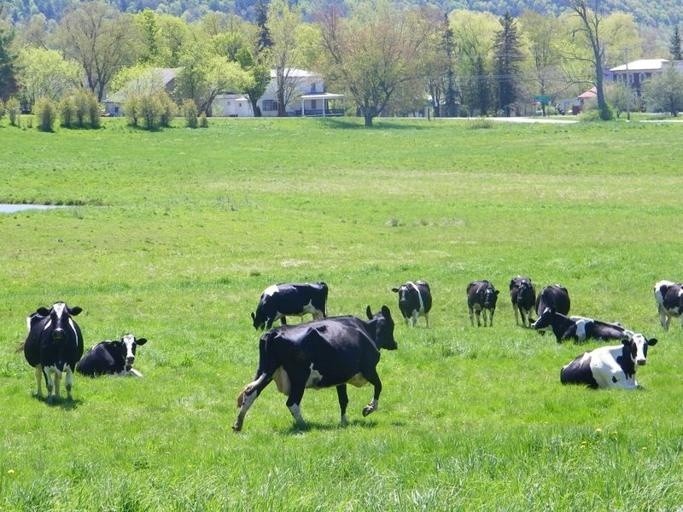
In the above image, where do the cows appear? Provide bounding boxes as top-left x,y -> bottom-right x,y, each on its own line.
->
20,300 -> 85,403
249,280 -> 330,333
77,334 -> 147,380
467,280 -> 500,327
391,279 -> 433,329
531,305 -> 637,346
530,283 -> 571,336
649,280 -> 683,332
231,303 -> 398,432
509,275 -> 536,327
560,332 -> 658,390
